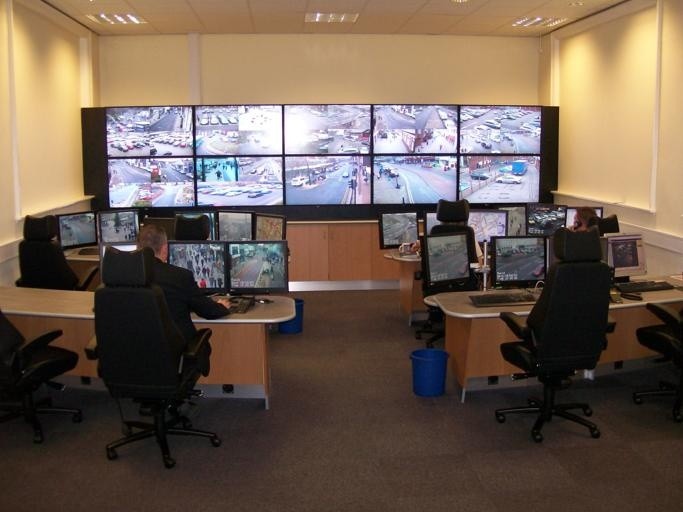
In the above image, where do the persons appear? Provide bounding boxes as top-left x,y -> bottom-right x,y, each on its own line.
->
136,226 -> 231,428
174,246 -> 226,286
568,208 -> 597,231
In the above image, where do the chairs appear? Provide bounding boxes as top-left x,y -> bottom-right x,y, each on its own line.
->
585,214 -> 620,237
632,303 -> 683,422
409,199 -> 482,347
0,307 -> 82,443
175,216 -> 210,241
13,214 -> 83,289
85,248 -> 223,469
495,225 -> 617,445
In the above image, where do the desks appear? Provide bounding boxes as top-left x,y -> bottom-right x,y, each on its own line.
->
422,271 -> 683,403
385,244 -> 434,327
63,245 -> 111,293
0,282 -> 295,412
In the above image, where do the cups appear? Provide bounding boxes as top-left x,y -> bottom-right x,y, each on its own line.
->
398,242 -> 411,254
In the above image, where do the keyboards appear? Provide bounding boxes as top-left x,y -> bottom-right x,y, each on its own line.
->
613,280 -> 674,294
209,294 -> 255,314
467,290 -> 539,307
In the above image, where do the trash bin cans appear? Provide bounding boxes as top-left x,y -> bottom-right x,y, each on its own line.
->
409,348 -> 448,397
278,298 -> 304,334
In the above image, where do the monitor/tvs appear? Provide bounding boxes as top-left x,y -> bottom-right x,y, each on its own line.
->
425,212 -> 443,238
165,239 -> 228,293
373,155 -> 459,206
95,208 -> 142,247
459,104 -> 543,156
57,210 -> 99,250
284,155 -> 372,205
214,210 -> 253,240
372,103 -> 459,155
565,204 -> 606,242
255,213 -> 287,242
488,234 -> 550,292
467,209 -> 508,242
227,240 -> 288,294
376,209 -> 422,250
422,236 -> 475,291
283,103 -> 372,155
523,205 -> 567,238
105,159 -> 197,211
195,157 -> 285,208
459,155 -> 542,206
193,104 -> 285,155
106,105 -> 195,158
604,232 -> 649,278
